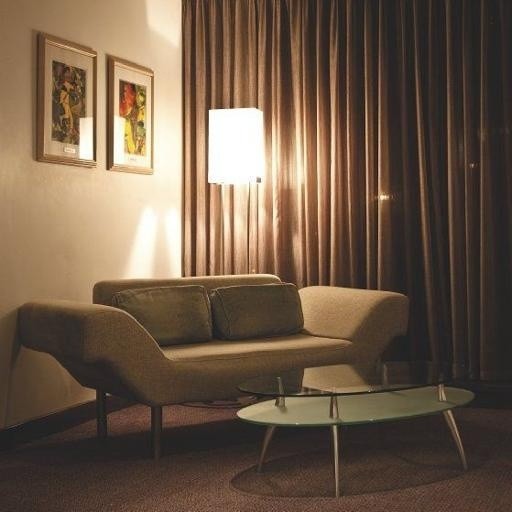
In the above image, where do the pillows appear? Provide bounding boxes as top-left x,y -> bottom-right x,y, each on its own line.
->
109,281 -> 304,345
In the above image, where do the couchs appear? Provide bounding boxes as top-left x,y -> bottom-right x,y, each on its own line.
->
16,272 -> 411,470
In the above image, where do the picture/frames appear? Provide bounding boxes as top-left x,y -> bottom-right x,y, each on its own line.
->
33,32 -> 156,177
